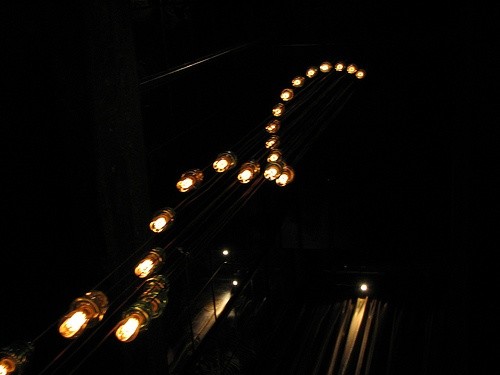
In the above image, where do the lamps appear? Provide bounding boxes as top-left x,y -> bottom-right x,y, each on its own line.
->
0,46 -> 403,375
